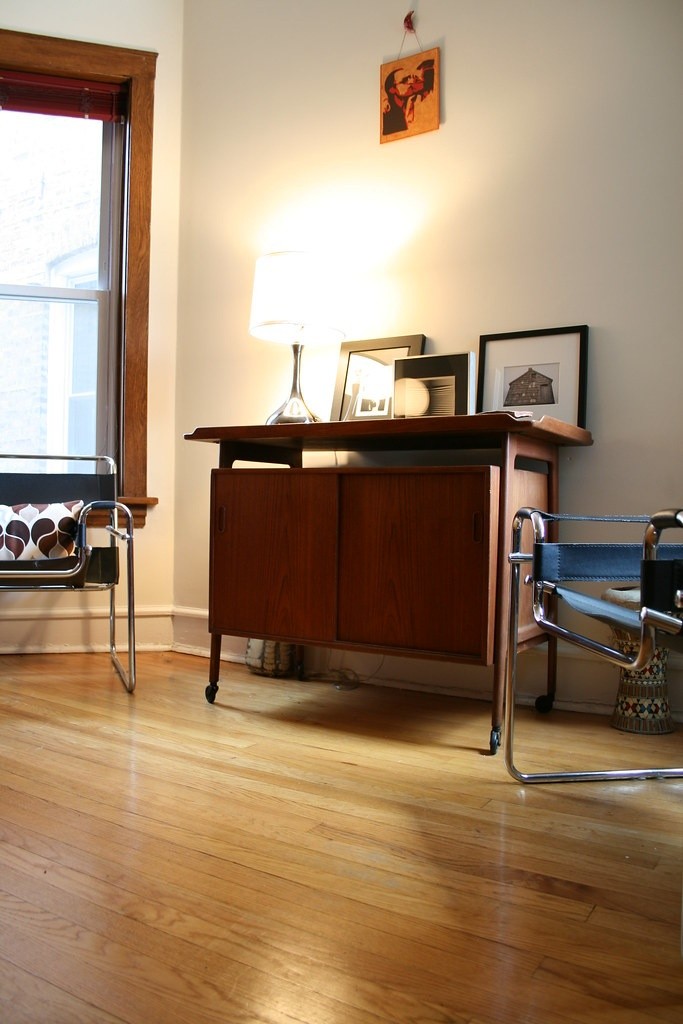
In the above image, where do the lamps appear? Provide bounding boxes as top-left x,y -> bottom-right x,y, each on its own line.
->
249,251 -> 337,425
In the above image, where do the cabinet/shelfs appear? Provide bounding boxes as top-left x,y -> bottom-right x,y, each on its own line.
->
184,415 -> 594,754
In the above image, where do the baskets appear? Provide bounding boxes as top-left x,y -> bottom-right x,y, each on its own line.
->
244,638 -> 303,677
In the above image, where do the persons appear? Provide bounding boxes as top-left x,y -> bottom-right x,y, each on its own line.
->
382,58 -> 435,136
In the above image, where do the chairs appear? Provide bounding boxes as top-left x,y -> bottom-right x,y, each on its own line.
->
506,506 -> 682,785
0,455 -> 136,693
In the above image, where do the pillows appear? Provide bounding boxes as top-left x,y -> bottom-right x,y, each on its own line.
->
0,500 -> 83,561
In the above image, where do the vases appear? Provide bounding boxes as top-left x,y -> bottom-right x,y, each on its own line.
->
601,585 -> 683,734
245,636 -> 300,677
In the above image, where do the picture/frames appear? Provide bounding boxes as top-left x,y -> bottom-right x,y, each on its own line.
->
475,325 -> 589,429
331,334 -> 426,421
